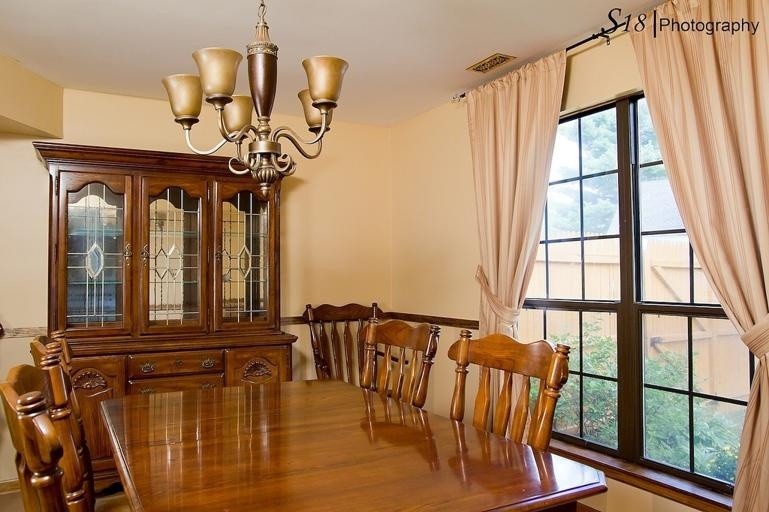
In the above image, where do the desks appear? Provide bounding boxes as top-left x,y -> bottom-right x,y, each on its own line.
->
100,379 -> 608,512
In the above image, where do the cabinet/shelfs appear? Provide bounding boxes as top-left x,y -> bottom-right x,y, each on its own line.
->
49,337 -> 128,495
208,157 -> 297,336
128,336 -> 225,396
32,140 -> 208,337
224,333 -> 298,386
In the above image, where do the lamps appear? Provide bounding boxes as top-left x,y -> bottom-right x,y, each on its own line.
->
162,0 -> 349,196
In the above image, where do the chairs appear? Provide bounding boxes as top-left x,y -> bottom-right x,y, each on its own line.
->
360,319 -> 440,408
0,364 -> 82,512
303,303 -> 384,385
448,331 -> 570,451
30,330 -> 131,512
